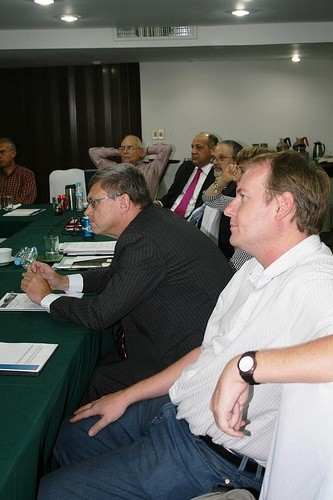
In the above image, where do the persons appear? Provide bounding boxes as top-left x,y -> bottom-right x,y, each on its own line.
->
37,149 -> 333,500
20,163 -> 238,410
21,133 -> 333,500
0,138 -> 37,205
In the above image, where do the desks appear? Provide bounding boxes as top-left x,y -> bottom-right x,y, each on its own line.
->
0,203 -> 116,500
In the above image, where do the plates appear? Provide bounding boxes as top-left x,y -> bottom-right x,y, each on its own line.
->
0,256 -> 15,266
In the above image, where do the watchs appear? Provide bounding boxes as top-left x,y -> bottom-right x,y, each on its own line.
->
237,350 -> 261,385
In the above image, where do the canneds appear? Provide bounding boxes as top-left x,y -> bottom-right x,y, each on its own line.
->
57,194 -> 67,211
82,216 -> 94,236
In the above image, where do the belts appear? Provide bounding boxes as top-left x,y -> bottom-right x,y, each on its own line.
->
196,435 -> 267,478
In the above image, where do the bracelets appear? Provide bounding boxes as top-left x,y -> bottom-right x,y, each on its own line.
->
212,176 -> 227,194
146,146 -> 151,155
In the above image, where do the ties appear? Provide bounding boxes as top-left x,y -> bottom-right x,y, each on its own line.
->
173,168 -> 203,218
116,330 -> 128,362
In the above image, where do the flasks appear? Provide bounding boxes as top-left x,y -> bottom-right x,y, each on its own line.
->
65,185 -> 76,210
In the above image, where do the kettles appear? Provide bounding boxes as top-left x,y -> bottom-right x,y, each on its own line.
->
314,142 -> 325,156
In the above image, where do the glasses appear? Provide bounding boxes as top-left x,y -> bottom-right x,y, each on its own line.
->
87,196 -> 110,208
0,150 -> 10,155
210,154 -> 232,161
119,145 -> 141,152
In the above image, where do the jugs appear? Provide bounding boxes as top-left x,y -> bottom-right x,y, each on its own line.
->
294,136 -> 309,152
277,137 -> 291,149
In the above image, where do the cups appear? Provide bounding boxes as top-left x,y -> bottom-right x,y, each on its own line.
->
4,195 -> 13,211
81,217 -> 92,237
0,248 -> 12,261
45,236 -> 59,258
52,197 -> 59,209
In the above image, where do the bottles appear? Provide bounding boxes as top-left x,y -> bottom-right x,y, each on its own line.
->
14,246 -> 39,269
76,182 -> 83,212
